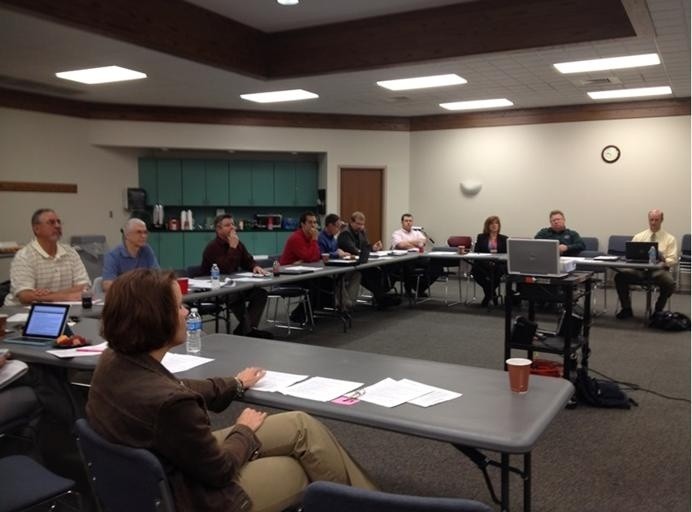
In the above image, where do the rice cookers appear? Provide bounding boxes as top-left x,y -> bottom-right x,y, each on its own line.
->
167,216 -> 180,231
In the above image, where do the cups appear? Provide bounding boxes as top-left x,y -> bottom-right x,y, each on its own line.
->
0,313 -> 7,338
320,252 -> 332,267
194,223 -> 215,231
177,279 -> 189,295
267,217 -> 273,231
505,358 -> 533,394
458,245 -> 466,255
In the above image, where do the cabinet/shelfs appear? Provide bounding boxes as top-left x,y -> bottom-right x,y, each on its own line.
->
236,231 -> 277,259
273,158 -> 319,207
137,157 -> 182,208
503,269 -> 595,384
181,159 -> 230,208
276,231 -> 295,257
183,230 -> 216,269
146,230 -> 184,272
230,159 -> 275,208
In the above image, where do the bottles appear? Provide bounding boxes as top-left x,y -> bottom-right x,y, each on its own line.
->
238,216 -> 256,231
489,237 -> 498,256
417,238 -> 424,256
210,262 -> 222,292
185,307 -> 204,355
283,215 -> 300,230
80,284 -> 95,317
648,246 -> 657,266
272,257 -> 281,277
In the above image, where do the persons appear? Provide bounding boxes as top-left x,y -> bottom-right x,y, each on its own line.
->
531,209 -> 586,310
3,207 -> 115,307
201,209 -> 390,338
100,218 -> 163,293
613,207 -> 677,322
467,214 -> 509,307
85,267 -> 380,512
390,212 -> 441,299
1,353 -> 41,424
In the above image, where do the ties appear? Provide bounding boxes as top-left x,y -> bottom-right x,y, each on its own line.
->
651,234 -> 656,242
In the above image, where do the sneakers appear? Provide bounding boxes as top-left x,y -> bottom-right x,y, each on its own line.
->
234,285 -> 497,337
616,308 -> 633,319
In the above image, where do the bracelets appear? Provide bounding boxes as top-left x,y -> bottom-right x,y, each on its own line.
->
234,375 -> 244,395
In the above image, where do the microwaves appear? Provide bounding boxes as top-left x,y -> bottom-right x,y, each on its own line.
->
255,213 -> 282,229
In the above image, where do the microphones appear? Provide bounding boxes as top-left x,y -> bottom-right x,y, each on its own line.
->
412,226 -> 424,231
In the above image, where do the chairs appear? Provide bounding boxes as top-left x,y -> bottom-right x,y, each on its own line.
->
303,480 -> 491,512
581,236 -> 600,252
0,247 -> 424,334
74,417 -> 176,511
608,235 -> 636,256
678,233 -> 691,291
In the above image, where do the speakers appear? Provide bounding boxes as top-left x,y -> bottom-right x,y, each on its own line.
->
510,314 -> 538,348
556,310 -> 584,340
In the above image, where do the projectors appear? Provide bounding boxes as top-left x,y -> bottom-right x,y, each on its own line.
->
558,259 -> 577,273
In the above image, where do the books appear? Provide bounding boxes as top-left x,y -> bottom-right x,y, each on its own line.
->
1,348 -> 29,391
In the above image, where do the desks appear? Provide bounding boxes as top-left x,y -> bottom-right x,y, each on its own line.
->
423,247 -> 673,324
0,315 -> 576,511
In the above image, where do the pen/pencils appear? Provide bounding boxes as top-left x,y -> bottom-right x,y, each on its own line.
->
74,348 -> 103,353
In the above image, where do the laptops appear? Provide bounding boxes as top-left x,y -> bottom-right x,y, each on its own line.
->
506,238 -> 569,278
626,242 -> 658,263
343,244 -> 370,263
4,303 -> 72,347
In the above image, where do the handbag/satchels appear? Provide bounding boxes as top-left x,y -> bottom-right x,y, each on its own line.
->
648,311 -> 691,331
576,368 -> 638,408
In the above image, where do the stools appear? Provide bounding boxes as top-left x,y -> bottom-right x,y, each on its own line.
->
0,453 -> 85,511
